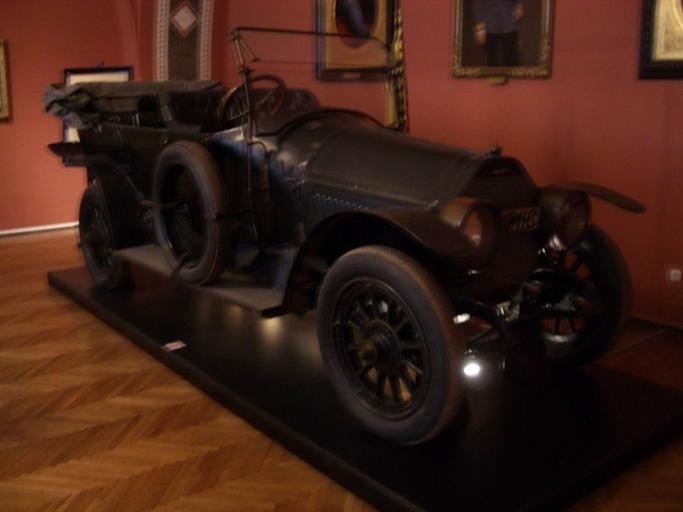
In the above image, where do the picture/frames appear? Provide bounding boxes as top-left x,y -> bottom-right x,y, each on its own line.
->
314,1 -> 402,83
450,0 -> 555,81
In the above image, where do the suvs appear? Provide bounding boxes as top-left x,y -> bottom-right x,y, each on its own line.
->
40,20 -> 650,457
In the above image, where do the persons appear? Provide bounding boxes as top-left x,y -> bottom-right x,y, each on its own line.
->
459,0 -> 530,69
